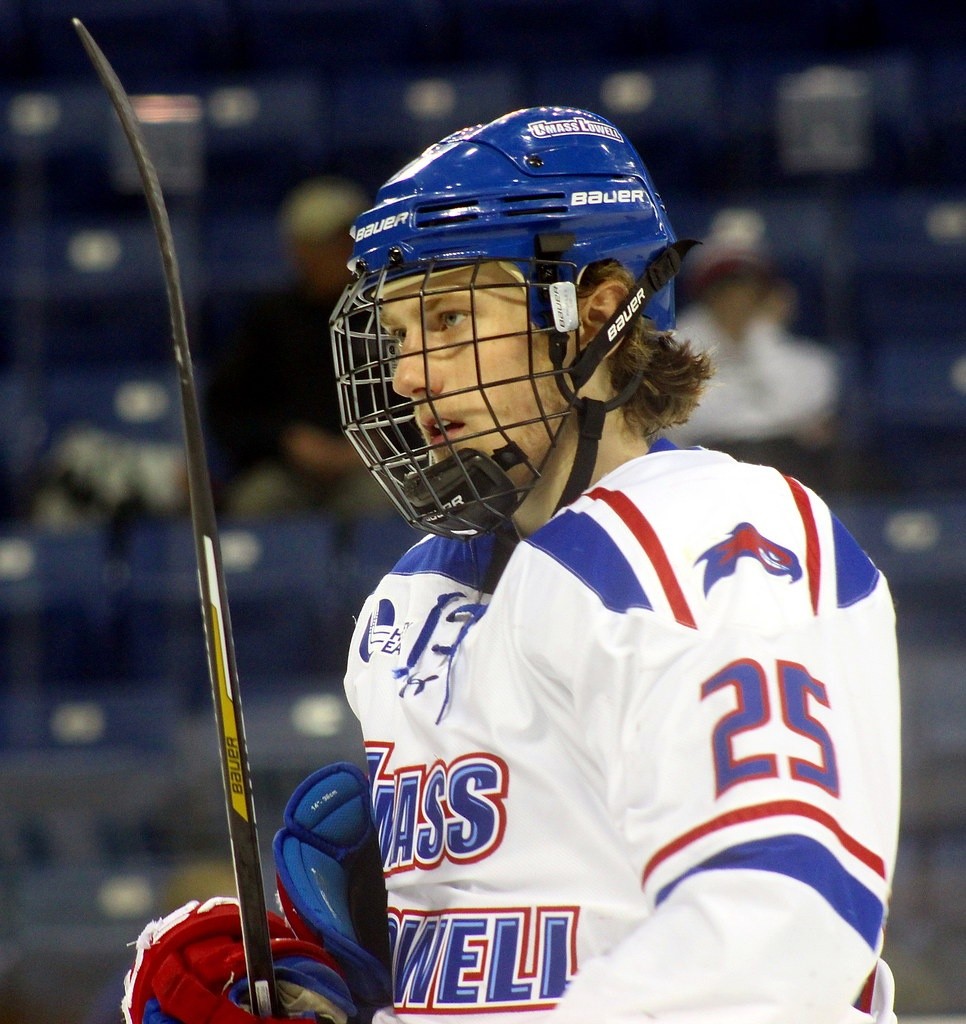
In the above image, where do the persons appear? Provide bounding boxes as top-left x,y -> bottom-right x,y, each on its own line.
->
208,190 -> 423,516
658,211 -> 838,491
121,105 -> 901,1024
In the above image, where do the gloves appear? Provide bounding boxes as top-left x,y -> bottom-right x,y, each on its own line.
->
119,894 -> 357,1023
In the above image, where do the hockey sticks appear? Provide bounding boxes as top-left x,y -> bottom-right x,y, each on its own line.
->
70,13 -> 280,1019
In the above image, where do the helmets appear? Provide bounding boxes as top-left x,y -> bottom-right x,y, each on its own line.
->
330,106 -> 680,542
